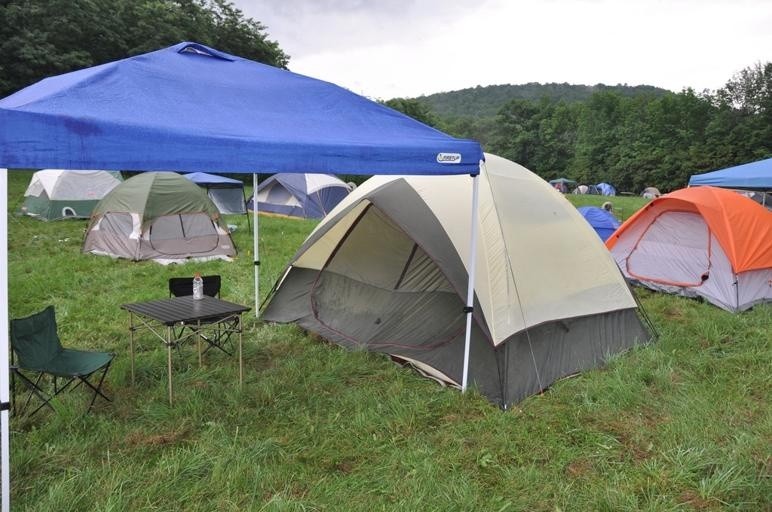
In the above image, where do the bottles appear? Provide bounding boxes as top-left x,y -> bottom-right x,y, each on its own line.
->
193,273 -> 203,300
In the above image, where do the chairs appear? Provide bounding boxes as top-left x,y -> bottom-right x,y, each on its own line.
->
168,275 -> 234,357
9,303 -> 117,419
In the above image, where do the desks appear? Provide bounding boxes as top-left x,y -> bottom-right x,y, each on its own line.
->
119,294 -> 253,409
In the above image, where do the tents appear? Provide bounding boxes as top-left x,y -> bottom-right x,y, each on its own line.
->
573,185 -> 589,194
687,157 -> 772,207
347,181 -> 357,190
605,186 -> 772,313
181,172 -> 251,236
577,206 -> 621,243
80,171 -> 238,262
549,177 -> 577,193
254,152 -> 660,412
592,182 -> 617,196
640,187 -> 661,199
247,174 -> 353,221
12,169 -> 125,223
0,41 -> 486,512
